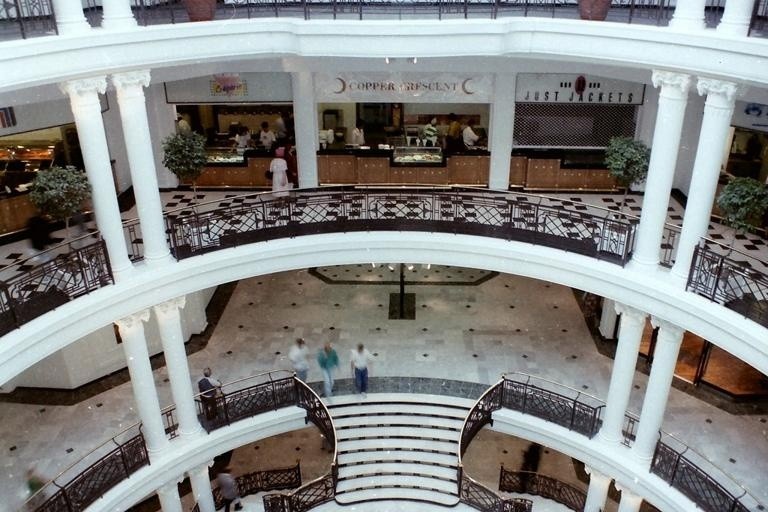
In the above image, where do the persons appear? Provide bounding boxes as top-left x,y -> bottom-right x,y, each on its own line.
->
27,469 -> 44,492
350,343 -> 376,394
315,341 -> 340,399
198,368 -> 223,422
445,113 -> 462,154
712,133 -> 768,227
176,116 -> 298,206
351,118 -> 366,146
462,119 -> 483,147
22,215 -> 69,302
217,466 -> 244,512
422,115 -> 441,147
288,338 -> 311,383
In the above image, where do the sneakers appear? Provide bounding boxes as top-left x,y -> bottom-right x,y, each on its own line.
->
234,506 -> 243,511
298,391 -> 368,404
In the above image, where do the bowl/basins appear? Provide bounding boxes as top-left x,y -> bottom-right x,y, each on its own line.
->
320,143 -> 329,150
384,127 -> 403,137
334,133 -> 343,142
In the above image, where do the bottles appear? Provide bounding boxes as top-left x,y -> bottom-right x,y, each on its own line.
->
352,128 -> 365,145
319,129 -> 334,144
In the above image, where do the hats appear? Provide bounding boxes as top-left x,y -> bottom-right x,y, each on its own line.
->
275,147 -> 285,157
431,117 -> 437,125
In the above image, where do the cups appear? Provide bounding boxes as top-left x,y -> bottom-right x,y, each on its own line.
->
378,145 -> 394,150
406,137 -> 435,147
